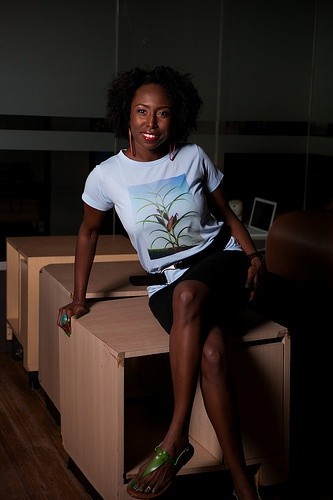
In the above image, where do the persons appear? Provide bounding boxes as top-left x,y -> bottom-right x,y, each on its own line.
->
57,66 -> 263,500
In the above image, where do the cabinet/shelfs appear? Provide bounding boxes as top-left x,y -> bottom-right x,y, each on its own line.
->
5,234 -> 292,500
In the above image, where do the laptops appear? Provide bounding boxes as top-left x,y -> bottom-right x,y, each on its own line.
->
244,197 -> 277,237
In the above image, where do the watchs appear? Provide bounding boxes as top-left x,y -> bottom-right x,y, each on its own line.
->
247,252 -> 260,263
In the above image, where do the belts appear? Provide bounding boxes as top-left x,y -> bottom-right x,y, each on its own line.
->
129,223 -> 231,287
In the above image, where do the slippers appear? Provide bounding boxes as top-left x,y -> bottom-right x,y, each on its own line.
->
127,444 -> 195,499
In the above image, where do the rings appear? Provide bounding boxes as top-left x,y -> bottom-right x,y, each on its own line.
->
63,317 -> 71,322
